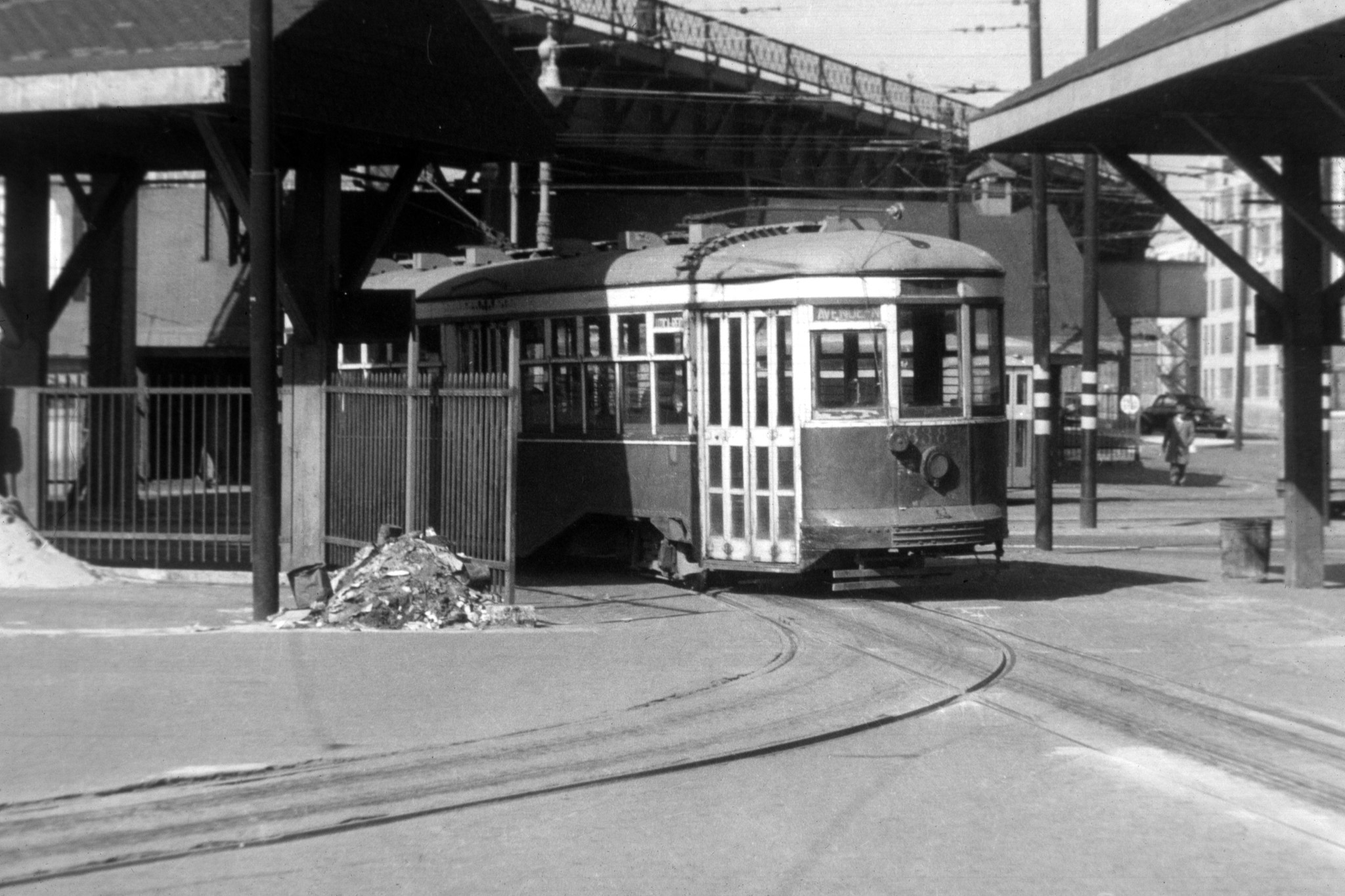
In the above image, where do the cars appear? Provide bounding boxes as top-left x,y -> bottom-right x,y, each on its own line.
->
1138,392 -> 1230,440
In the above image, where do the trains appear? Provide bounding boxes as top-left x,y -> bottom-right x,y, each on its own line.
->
413,214 -> 1011,596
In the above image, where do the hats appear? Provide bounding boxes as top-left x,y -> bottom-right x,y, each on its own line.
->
1174,405 -> 1190,415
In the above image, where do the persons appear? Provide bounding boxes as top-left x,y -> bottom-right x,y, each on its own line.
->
1162,404 -> 1196,486
